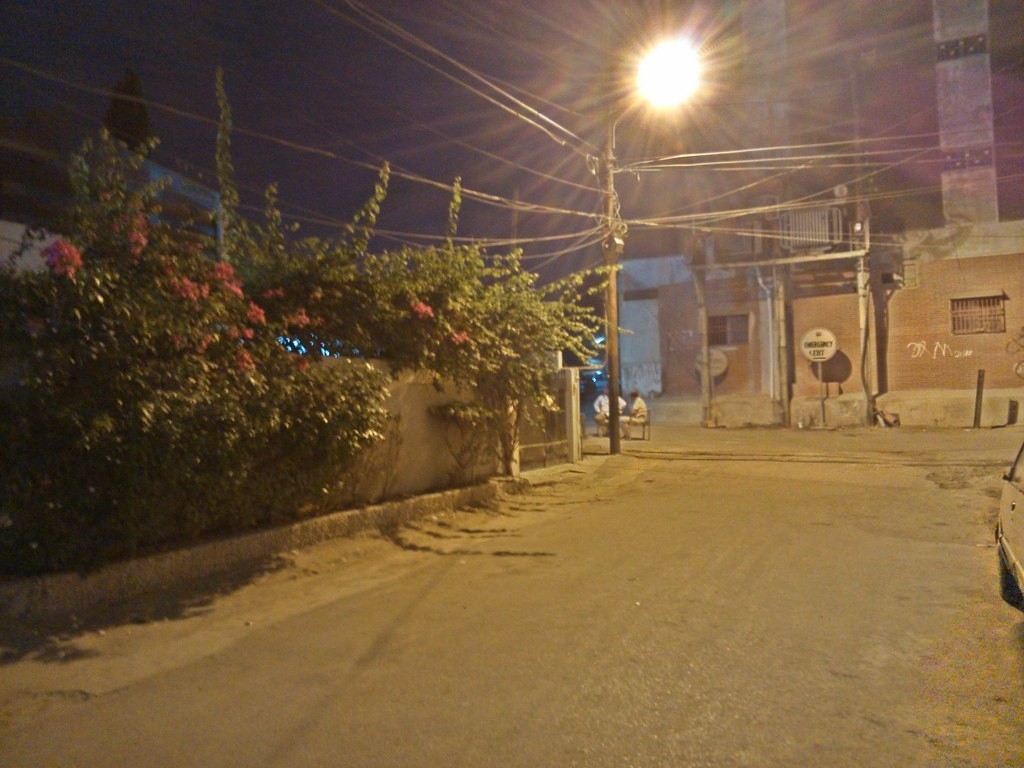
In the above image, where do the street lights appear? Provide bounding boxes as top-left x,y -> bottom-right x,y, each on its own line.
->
596,33 -> 709,454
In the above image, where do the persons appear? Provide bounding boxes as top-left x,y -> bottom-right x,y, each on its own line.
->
593,389 -> 627,437
619,392 -> 647,440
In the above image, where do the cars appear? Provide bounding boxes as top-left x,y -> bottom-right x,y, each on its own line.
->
994,440 -> 1024,599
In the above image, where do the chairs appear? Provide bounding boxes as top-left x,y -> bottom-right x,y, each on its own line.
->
629,410 -> 650,441
